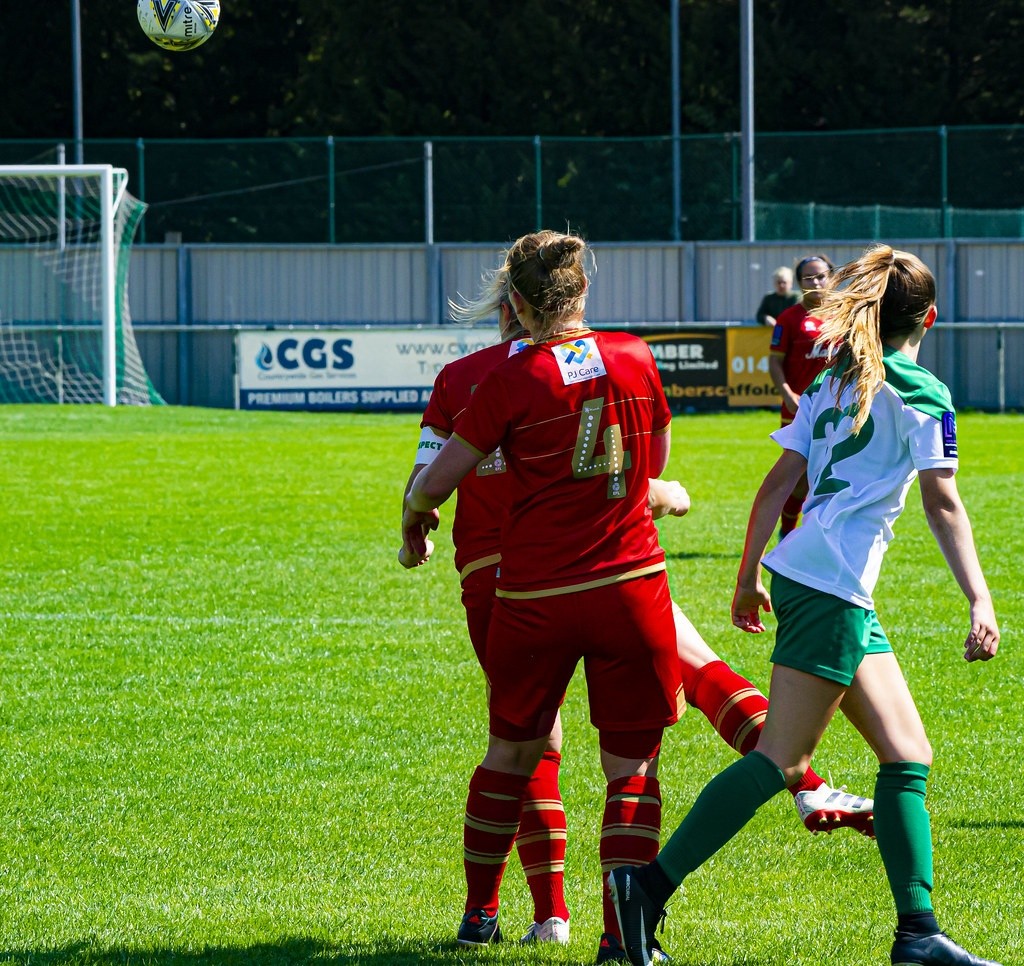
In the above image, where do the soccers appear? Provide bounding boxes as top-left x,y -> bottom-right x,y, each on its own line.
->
136,0 -> 222,53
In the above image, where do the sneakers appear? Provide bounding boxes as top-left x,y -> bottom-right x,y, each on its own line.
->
607,865 -> 653,966
597,932 -> 630,964
519,916 -> 569,944
794,782 -> 875,842
651,948 -> 673,962
890,929 -> 1004,966
456,909 -> 503,946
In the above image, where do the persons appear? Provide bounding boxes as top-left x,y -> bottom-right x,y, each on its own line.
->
403,231 -> 683,965
395,265 -> 878,945
770,255 -> 853,544
607,245 -> 1006,966
756,267 -> 800,327
